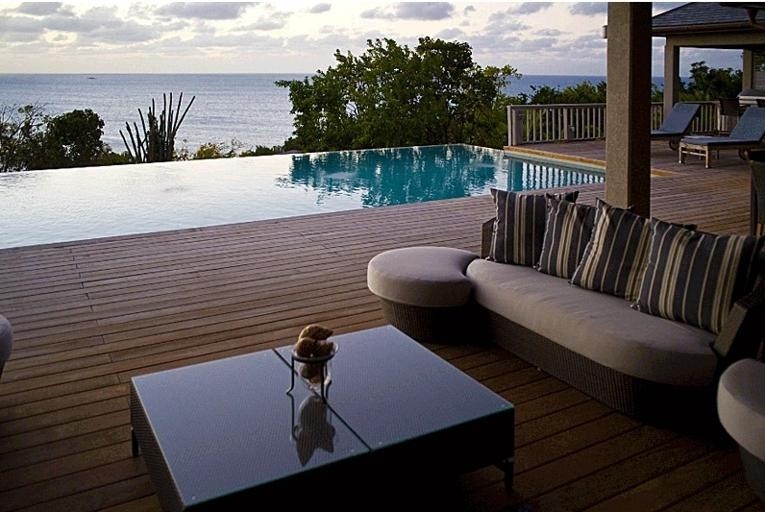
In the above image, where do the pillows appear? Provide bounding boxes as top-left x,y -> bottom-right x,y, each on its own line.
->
486,188 -> 757,335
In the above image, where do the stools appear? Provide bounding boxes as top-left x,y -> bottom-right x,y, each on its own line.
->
367,246 -> 480,342
717,356 -> 765,501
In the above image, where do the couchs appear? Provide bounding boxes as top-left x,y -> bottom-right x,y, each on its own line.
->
466,215 -> 765,422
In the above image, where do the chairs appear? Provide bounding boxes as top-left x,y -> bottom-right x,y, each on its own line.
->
650,103 -> 765,169
